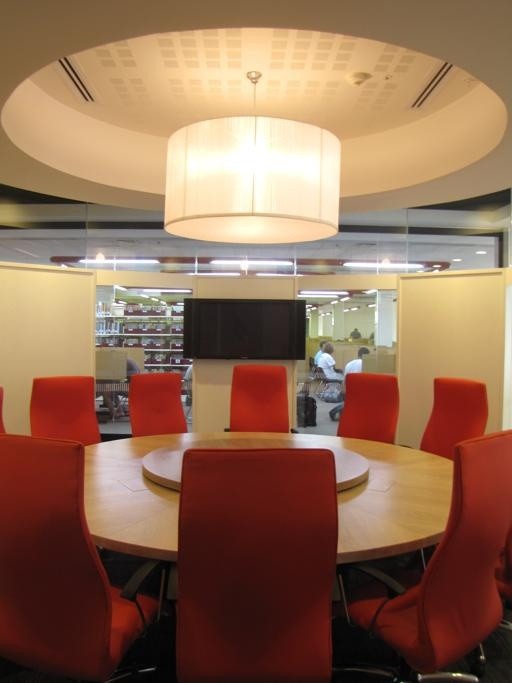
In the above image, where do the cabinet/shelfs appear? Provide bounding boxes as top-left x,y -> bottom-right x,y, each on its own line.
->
95,313 -> 193,368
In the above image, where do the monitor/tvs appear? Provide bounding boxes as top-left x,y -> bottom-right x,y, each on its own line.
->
184,296 -> 307,360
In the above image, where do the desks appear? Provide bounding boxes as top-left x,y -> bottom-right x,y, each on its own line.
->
83,429 -> 457,567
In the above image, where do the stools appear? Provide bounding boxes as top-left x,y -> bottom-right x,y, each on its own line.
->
111,391 -> 128,421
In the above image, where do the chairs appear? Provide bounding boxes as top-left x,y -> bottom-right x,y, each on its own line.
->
347,431 -> 512,683
0,433 -> 170,682
308,357 -> 342,400
29,376 -> 102,446
336,374 -> 400,445
175,447 -> 338,683
127,373 -> 188,437
0,387 -> 6,435
229,365 -> 289,433
419,377 -> 488,460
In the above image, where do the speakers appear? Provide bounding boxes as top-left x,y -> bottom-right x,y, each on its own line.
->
297,396 -> 316,426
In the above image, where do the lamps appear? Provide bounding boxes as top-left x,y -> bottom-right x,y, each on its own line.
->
162,70 -> 342,246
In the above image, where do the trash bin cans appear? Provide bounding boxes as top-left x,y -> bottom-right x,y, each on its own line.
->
296,396 -> 317,428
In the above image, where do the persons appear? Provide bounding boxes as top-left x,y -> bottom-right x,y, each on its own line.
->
350,328 -> 361,339
344,347 -> 370,386
318,343 -> 345,380
314,341 -> 328,366
103,357 -> 141,419
185,364 -> 193,397
